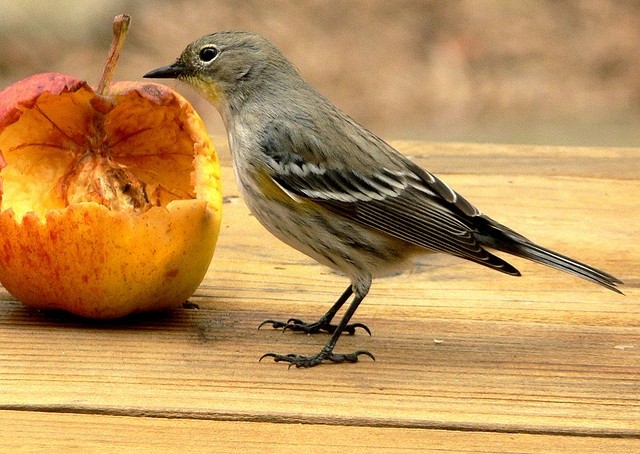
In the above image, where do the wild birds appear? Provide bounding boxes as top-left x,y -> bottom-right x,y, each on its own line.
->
143,29 -> 627,370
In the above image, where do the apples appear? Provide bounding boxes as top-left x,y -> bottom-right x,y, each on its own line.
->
0,15 -> 221,321
